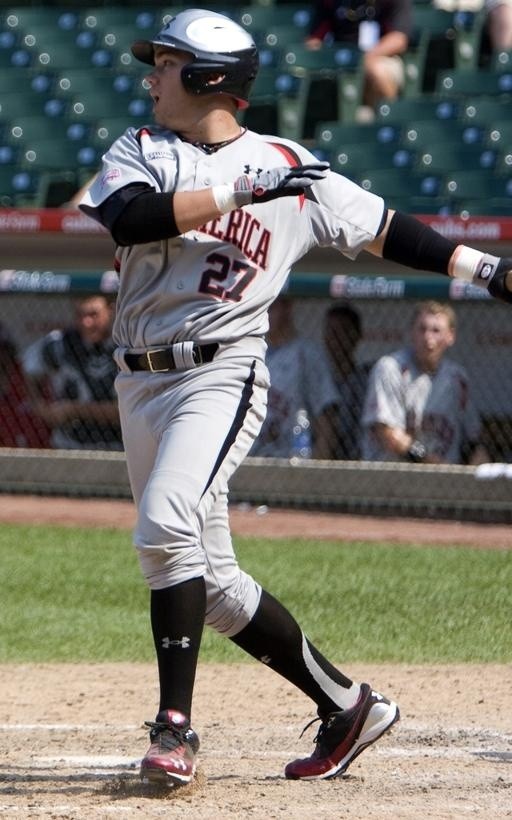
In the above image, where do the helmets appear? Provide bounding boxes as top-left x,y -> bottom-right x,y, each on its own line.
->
132,8 -> 259,111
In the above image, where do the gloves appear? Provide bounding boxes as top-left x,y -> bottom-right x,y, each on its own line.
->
473,252 -> 512,299
234,161 -> 328,208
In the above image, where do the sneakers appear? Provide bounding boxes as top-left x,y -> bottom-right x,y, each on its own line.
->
142,711 -> 200,788
286,683 -> 400,780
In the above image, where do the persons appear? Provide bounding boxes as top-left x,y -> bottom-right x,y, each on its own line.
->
287,0 -> 512,123
74,7 -> 512,791
1,290 -> 124,452
246,296 -> 491,466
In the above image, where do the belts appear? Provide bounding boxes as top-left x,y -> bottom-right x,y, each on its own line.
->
126,345 -> 217,374
